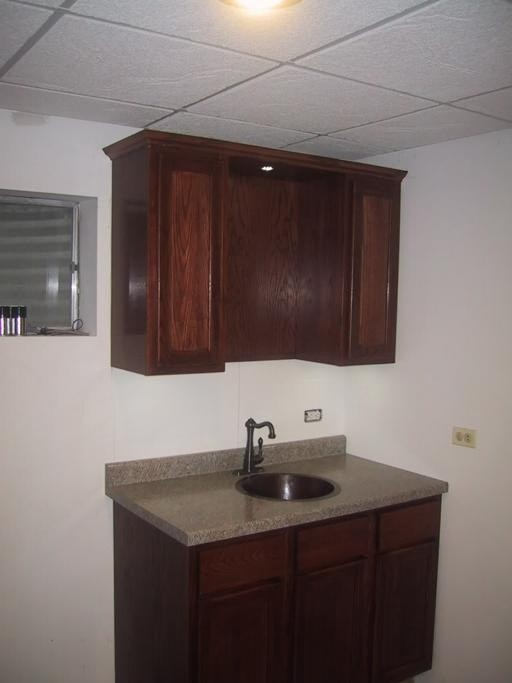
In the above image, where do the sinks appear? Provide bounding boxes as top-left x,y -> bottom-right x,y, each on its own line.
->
235,472 -> 341,503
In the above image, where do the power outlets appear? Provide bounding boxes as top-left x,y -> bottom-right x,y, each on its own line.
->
450,426 -> 477,448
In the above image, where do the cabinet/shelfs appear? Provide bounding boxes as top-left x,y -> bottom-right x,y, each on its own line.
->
102,127 -> 410,377
112,493 -> 443,683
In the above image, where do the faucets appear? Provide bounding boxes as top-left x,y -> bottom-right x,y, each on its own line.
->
244,419 -> 276,472
